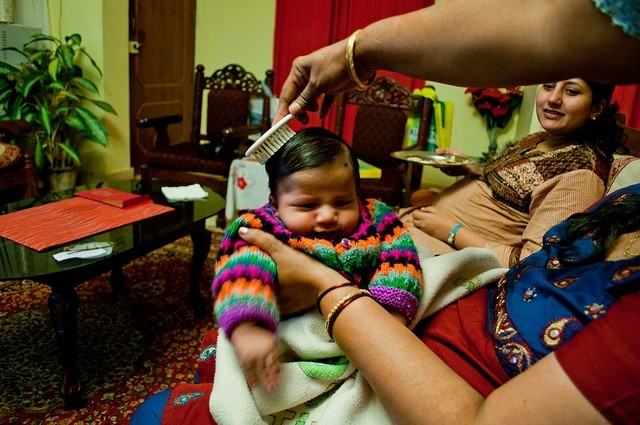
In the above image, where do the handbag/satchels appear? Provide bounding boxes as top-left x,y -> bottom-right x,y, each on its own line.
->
345,29 -> 376,92
315,281 -> 359,316
325,289 -> 372,342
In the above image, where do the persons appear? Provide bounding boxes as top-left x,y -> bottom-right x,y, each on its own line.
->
270,0 -> 639,128
212,125 -> 425,394
127,182 -> 640,425
397,76 -> 615,267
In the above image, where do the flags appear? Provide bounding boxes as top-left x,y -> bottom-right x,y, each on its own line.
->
485,128 -> 499,154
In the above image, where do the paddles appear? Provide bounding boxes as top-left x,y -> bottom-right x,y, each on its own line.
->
0,226 -> 225,424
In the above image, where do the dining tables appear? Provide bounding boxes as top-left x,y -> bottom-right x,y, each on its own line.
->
1,30 -> 119,194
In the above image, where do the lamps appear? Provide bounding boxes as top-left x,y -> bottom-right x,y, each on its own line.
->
135,62 -> 273,227
0,119 -> 39,205
334,76 -> 437,207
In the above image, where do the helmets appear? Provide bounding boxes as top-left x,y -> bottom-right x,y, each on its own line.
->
390,150 -> 472,167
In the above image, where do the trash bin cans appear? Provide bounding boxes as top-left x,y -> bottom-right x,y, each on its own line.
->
447,224 -> 463,246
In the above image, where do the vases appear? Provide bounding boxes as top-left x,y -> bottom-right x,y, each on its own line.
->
464,85 -> 524,129
